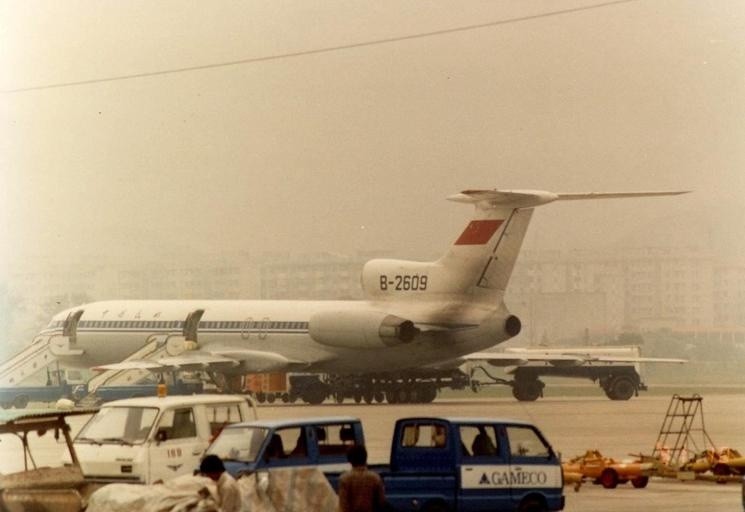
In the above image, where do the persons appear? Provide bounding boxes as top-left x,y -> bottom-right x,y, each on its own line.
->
197,455 -> 242,512
337,445 -> 387,512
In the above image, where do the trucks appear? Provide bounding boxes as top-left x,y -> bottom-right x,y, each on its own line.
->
326,416 -> 566,511
64,394 -> 259,495
193,417 -> 389,496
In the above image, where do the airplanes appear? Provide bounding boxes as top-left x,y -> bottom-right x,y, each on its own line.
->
0,187 -> 692,404
